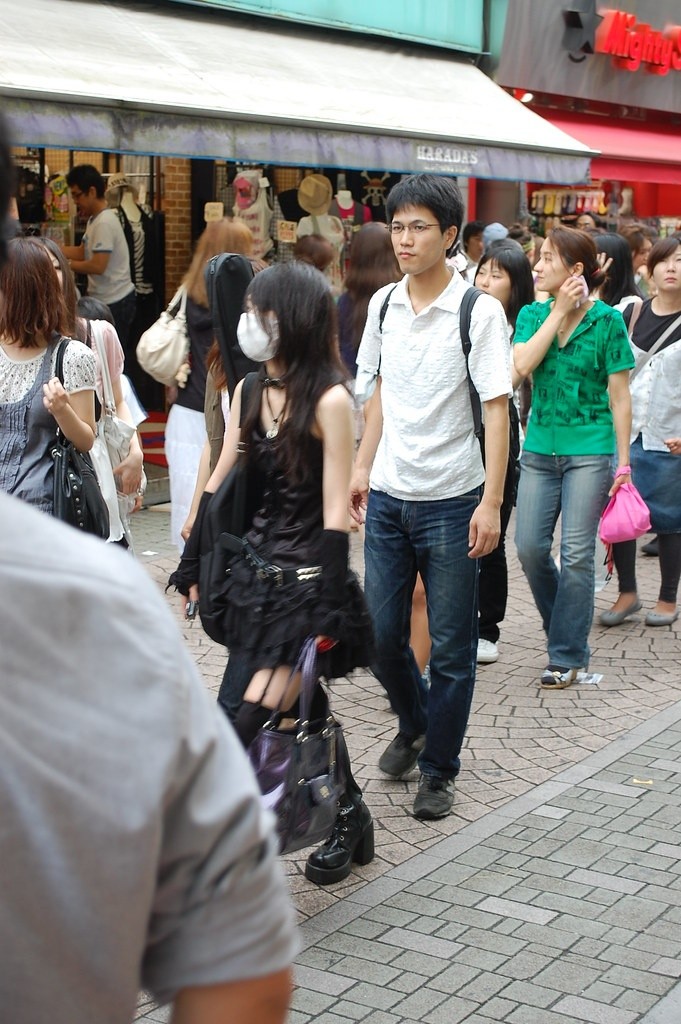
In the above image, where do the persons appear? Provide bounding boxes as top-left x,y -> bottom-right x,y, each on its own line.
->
0,482 -> 302,1024
294,208 -> 347,305
109,190 -> 165,409
327,187 -> 372,227
163,208 -> 435,677
349,174 -> 517,819
167,262 -> 378,885
446,208 -> 681,691
0,153 -> 148,557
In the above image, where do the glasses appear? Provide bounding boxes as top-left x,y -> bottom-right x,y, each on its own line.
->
71,188 -> 88,198
385,220 -> 440,234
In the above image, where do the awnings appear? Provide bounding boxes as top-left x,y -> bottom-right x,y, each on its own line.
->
0,0 -> 600,192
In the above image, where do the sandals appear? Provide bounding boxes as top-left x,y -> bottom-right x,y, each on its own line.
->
541,667 -> 576,689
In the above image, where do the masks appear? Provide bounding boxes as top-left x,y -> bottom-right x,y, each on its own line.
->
237,313 -> 281,362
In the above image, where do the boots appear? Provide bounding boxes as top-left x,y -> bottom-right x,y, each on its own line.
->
305,722 -> 375,885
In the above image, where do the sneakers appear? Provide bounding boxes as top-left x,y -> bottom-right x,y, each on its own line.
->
413,774 -> 456,818
379,732 -> 425,775
476,638 -> 498,662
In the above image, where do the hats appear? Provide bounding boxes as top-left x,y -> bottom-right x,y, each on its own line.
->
103,173 -> 139,206
298,174 -> 333,215
233,169 -> 259,208
483,223 -> 508,246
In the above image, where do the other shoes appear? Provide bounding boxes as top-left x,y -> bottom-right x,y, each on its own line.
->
644,607 -> 679,625
601,597 -> 642,624
642,537 -> 659,555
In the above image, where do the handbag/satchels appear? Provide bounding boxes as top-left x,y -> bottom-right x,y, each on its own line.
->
135,285 -> 189,387
248,634 -> 343,852
90,319 -> 138,483
49,338 -> 110,539
598,467 -> 653,543
196,460 -> 239,646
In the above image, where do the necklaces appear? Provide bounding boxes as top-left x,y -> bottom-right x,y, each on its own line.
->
265,386 -> 288,440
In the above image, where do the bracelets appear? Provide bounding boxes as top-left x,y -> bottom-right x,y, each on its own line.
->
67,259 -> 72,265
